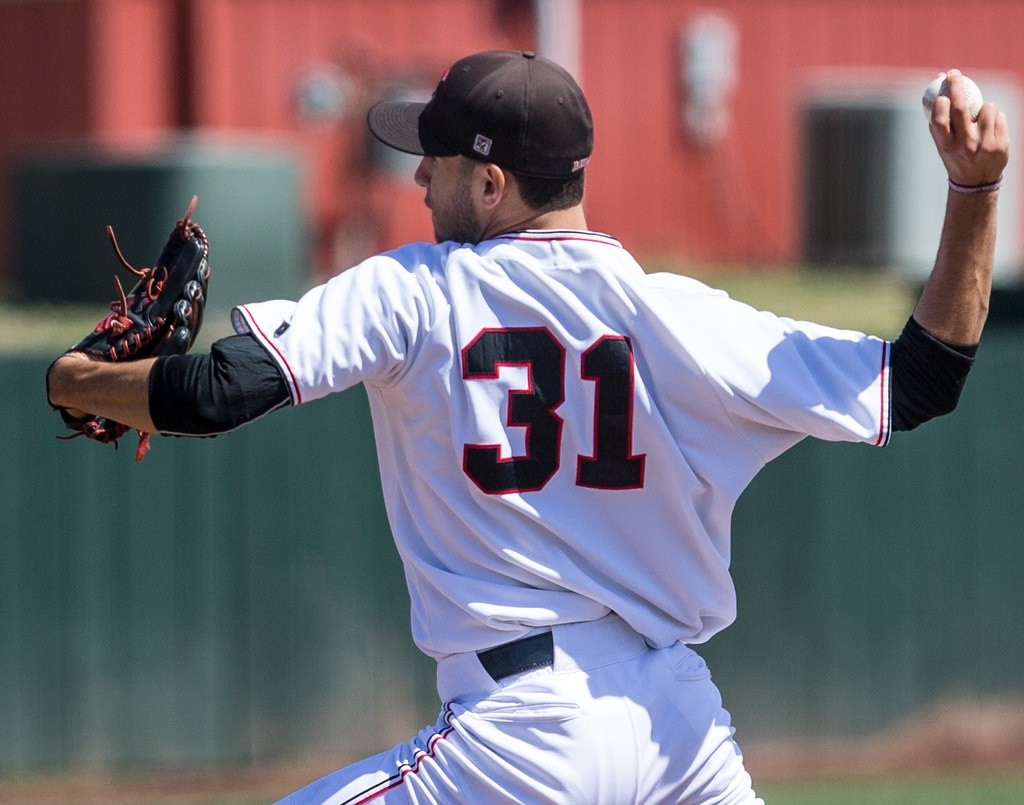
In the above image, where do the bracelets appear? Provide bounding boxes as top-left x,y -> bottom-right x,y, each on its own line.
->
948,175 -> 1004,191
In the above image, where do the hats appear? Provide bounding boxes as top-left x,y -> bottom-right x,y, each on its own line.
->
369,49 -> 594,177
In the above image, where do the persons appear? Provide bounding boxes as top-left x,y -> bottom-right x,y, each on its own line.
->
45,50 -> 1011,805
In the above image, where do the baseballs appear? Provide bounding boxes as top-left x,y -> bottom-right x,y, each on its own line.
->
920,74 -> 983,124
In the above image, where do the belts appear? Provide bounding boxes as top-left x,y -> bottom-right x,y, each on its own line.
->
478,631 -> 554,682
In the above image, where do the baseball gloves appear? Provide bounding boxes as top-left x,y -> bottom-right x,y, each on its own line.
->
47,219 -> 210,446
